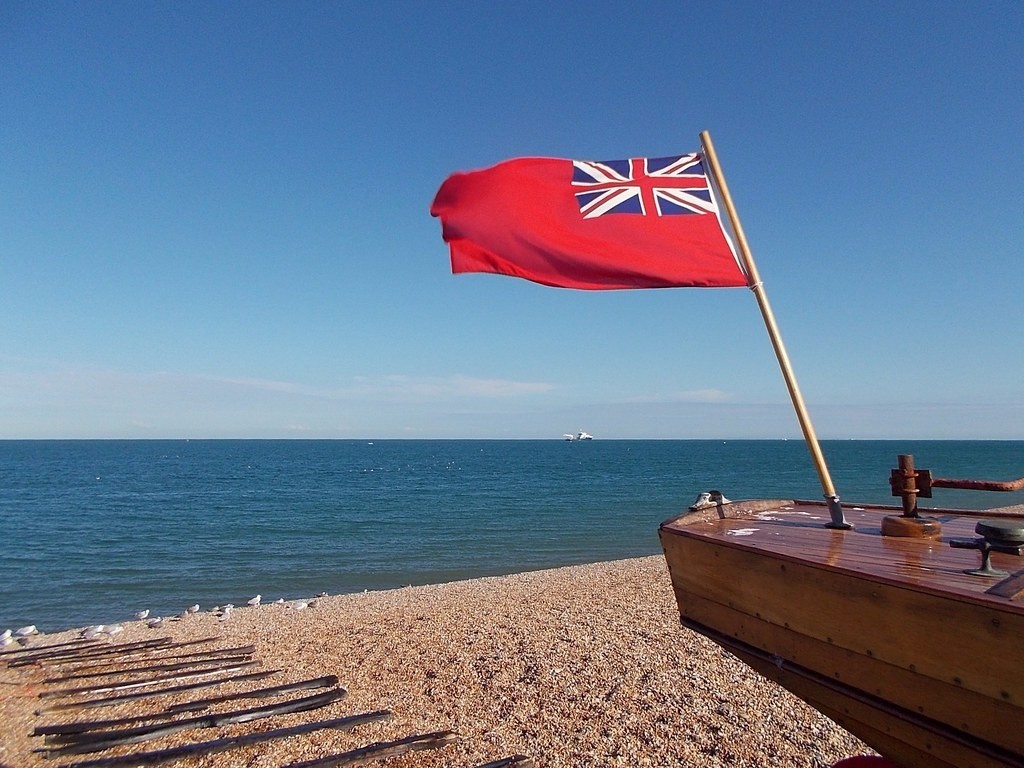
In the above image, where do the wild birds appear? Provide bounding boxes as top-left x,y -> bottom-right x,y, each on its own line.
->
0,592 -> 329,649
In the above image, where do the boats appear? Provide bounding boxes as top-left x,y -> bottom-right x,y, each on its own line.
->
562,431 -> 594,443
655,129 -> 1024,768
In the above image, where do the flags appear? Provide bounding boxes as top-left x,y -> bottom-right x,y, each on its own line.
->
429,152 -> 752,290
